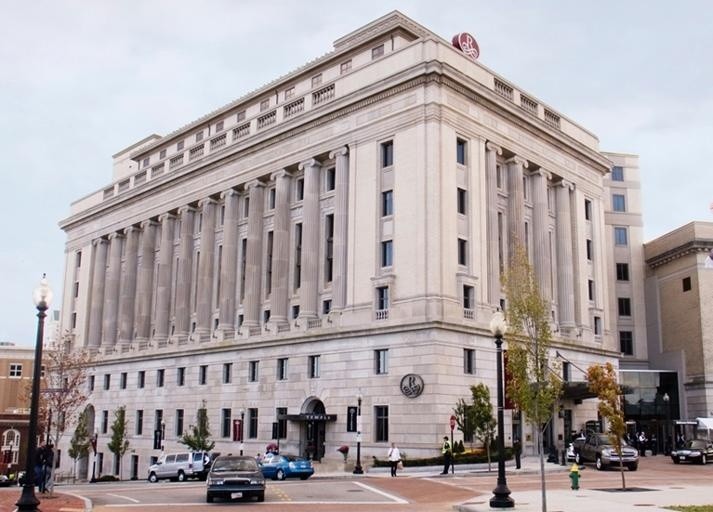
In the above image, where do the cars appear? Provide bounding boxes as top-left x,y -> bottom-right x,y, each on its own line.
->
258,452 -> 313,481
204,455 -> 264,501
670,438 -> 713,463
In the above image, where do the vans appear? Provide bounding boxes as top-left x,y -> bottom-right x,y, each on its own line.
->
146,451 -> 208,481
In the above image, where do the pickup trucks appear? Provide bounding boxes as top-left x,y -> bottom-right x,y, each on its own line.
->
573,433 -> 639,470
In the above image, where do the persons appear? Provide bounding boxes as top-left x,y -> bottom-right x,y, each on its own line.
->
254,452 -> 261,464
452,440 -> 458,452
457,440 -> 465,452
388,442 -> 400,477
512,437 -> 522,469
439,436 -> 452,474
202,453 -> 210,470
625,431 -> 686,457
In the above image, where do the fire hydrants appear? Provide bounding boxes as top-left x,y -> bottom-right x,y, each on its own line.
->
568,462 -> 581,490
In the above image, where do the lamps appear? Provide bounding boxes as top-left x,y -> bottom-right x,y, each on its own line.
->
559,409 -> 566,418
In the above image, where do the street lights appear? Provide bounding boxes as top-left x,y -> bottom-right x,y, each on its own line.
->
488,309 -> 515,507
14,272 -> 55,512
353,393 -> 363,473
